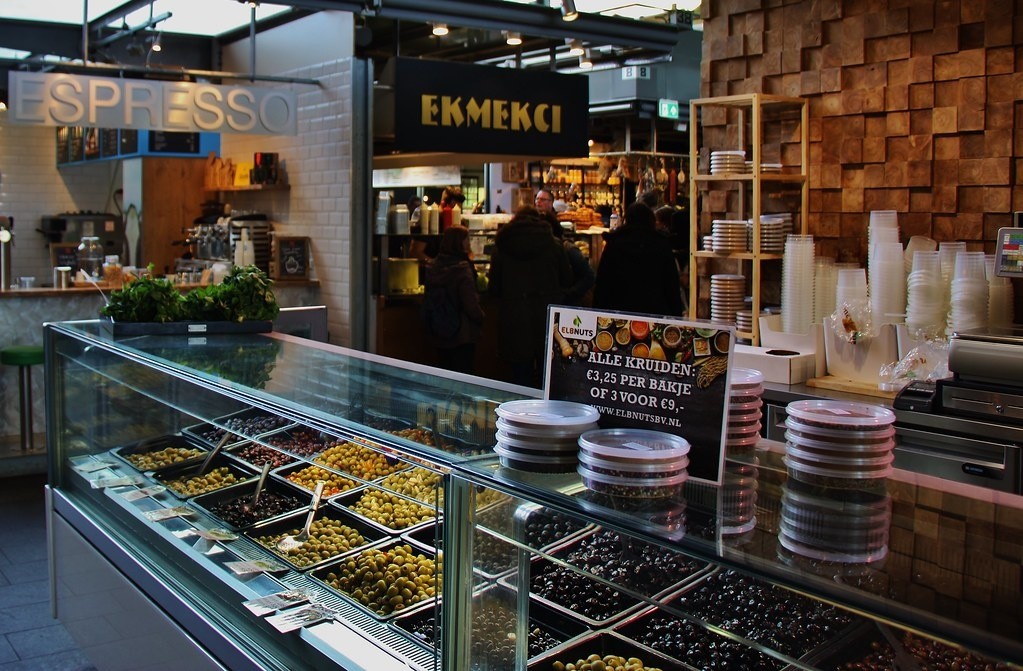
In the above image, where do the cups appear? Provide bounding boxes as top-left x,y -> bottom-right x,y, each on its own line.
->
54,267 -> 71,289
781,211 -> 1016,337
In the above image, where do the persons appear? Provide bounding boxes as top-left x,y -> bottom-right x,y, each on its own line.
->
408,196 -> 422,218
442,186 -> 466,212
652,205 -> 677,234
535,208 -> 596,304
594,202 -> 684,321
535,189 -> 559,222
485,201 -> 588,392
670,209 -> 690,318
421,222 -> 483,380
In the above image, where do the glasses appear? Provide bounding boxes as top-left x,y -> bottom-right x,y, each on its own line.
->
535,197 -> 551,201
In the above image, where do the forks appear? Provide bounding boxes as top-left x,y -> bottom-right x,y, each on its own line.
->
616,320 -> 630,345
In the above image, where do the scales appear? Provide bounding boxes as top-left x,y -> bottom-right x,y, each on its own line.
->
946,226 -> 1023,384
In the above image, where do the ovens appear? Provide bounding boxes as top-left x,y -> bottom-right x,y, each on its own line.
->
37,210 -> 123,271
171,213 -> 272,282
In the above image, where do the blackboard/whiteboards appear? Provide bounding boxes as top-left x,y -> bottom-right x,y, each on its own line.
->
275,235 -> 311,281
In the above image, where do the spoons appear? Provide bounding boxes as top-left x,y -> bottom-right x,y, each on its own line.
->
277,481 -> 324,552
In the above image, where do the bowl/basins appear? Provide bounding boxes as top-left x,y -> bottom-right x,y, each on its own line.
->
595,331 -> 614,352
662,326 -> 682,349
632,343 -> 649,359
714,331 -> 730,354
630,320 -> 649,340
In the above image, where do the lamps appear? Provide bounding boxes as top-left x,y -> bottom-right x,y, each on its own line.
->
578,45 -> 593,70
431,21 -> 449,37
504,31 -> 522,46
566,37 -> 584,58
560,0 -> 579,21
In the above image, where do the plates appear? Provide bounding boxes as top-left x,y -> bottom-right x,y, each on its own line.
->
695,327 -> 718,338
693,338 -> 711,356
107,404 -> 1023,671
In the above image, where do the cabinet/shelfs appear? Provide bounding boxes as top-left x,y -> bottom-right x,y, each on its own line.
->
41,311 -> 1022,670
685,91 -> 812,349
369,221 -> 614,384
524,158 -> 687,225
186,150 -> 292,279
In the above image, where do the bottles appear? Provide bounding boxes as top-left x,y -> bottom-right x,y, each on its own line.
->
452,204 -> 461,225
420,203 -> 429,235
610,211 -> 618,231
430,201 -> 439,235
443,205 -> 452,233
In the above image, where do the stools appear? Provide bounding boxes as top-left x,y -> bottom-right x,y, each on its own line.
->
0,345 -> 44,451
